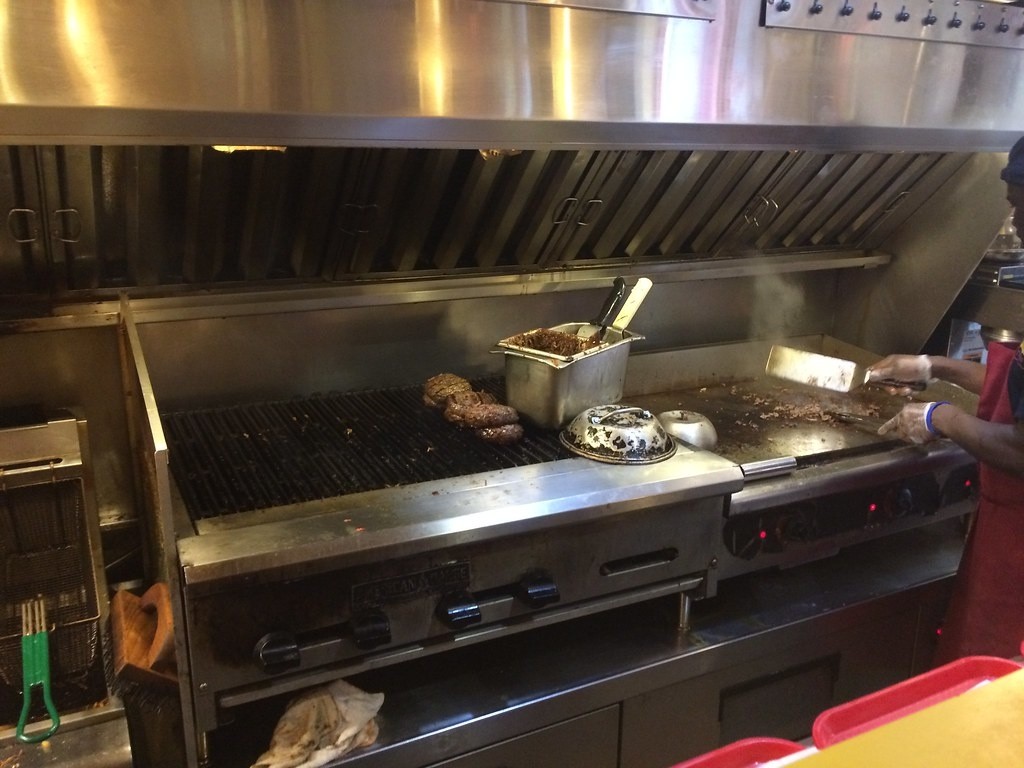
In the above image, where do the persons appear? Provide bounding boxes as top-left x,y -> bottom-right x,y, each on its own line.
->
865,136 -> 1024,672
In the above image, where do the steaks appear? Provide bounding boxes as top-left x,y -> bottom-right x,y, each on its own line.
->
424,373 -> 524,444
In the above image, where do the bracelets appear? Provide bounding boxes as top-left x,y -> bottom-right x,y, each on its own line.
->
925,401 -> 951,436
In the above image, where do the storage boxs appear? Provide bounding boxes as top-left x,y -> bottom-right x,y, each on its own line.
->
488,321 -> 646,430
810,655 -> 1024,750
668,736 -> 806,768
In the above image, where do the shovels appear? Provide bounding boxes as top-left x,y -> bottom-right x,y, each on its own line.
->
602,277 -> 653,343
764,344 -> 926,394
576,276 -> 626,342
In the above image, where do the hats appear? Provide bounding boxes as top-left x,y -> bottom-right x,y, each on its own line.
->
1000,137 -> 1024,185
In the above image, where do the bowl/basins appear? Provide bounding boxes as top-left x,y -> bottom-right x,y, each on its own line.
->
488,319 -> 646,429
984,248 -> 1024,264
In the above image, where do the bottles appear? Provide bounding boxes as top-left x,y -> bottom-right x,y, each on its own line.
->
994,216 -> 1017,250
